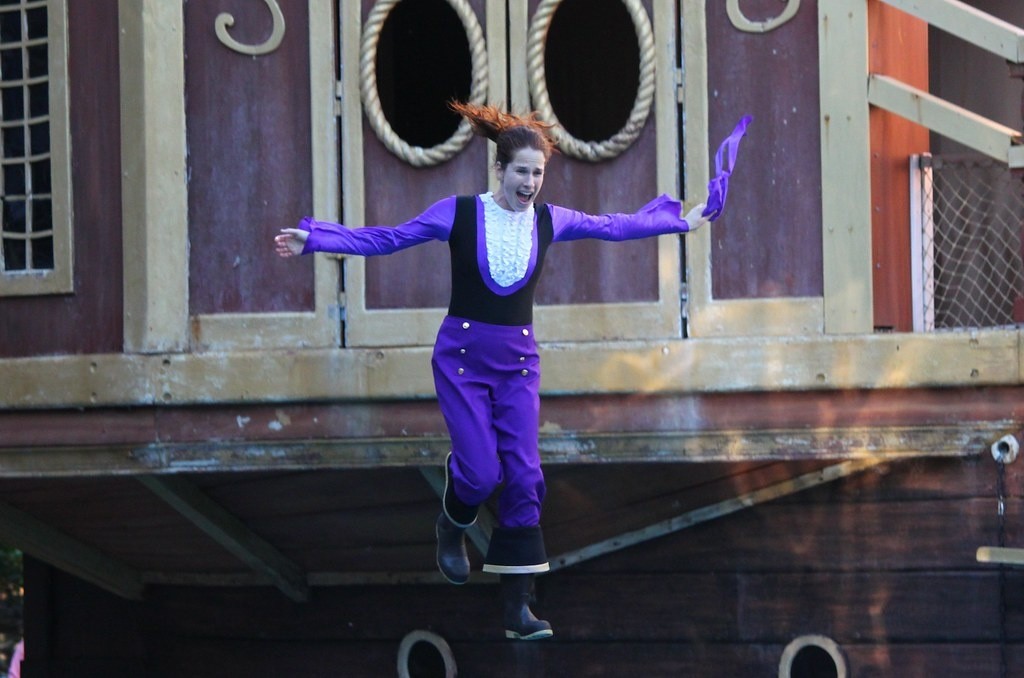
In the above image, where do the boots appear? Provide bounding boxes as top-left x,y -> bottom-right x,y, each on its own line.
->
482,526 -> 554,640
435,453 -> 479,584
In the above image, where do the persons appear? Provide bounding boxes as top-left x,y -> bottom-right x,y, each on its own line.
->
275,102 -> 720,641
